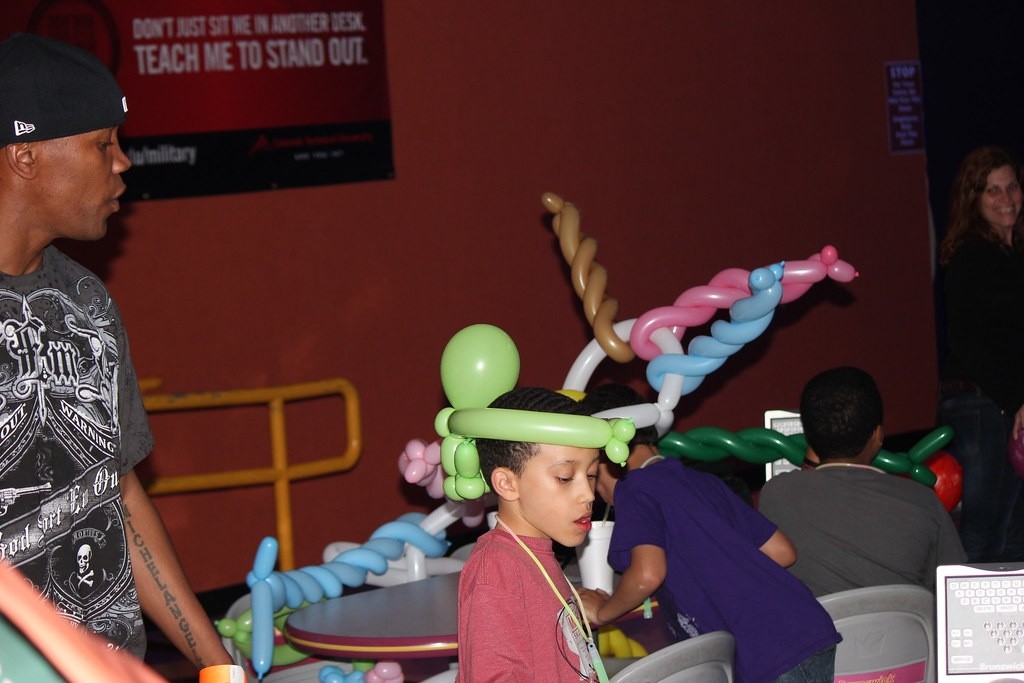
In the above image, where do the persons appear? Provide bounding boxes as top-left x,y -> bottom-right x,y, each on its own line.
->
457,388 -> 601,683
759,366 -> 969,597
0,33 -> 247,683
935,146 -> 1024,563
576,381 -> 843,683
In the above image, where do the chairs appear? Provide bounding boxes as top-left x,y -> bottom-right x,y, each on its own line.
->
609,630 -> 736,683
816,584 -> 937,683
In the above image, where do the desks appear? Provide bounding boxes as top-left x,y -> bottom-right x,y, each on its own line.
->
284,571 -> 458,660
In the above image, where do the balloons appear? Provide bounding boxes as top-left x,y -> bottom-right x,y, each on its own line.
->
212,193 -> 965,682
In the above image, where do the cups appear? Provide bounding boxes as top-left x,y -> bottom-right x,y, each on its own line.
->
577,519 -> 615,595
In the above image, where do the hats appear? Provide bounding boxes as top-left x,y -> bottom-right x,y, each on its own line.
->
0,34 -> 129,150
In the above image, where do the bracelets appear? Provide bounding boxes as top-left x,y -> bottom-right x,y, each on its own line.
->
197,665 -> 247,683
803,458 -> 820,466
